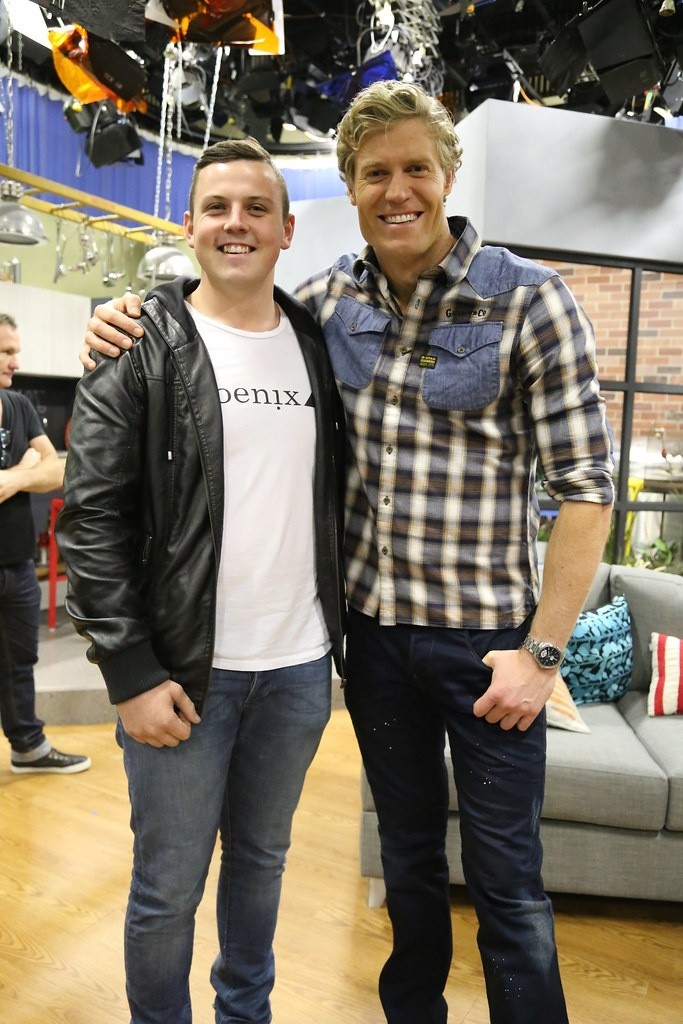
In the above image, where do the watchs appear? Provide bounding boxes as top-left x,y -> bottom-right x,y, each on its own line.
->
522,633 -> 566,670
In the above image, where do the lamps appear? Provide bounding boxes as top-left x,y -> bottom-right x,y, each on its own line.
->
465,76 -> 521,112
137,232 -> 196,283
0,182 -> 49,245
64,101 -> 141,169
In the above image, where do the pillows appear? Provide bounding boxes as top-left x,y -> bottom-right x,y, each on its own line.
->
547,593 -> 634,732
647,632 -> 682,718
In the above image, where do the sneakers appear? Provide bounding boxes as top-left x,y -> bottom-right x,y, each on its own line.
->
10,746 -> 91,774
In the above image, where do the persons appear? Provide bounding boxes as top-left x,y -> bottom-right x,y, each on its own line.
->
78,79 -> 617,1024
0,313 -> 92,775
53,138 -> 347,1024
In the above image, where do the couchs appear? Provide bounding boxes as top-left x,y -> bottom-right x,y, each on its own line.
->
358,537 -> 683,908
